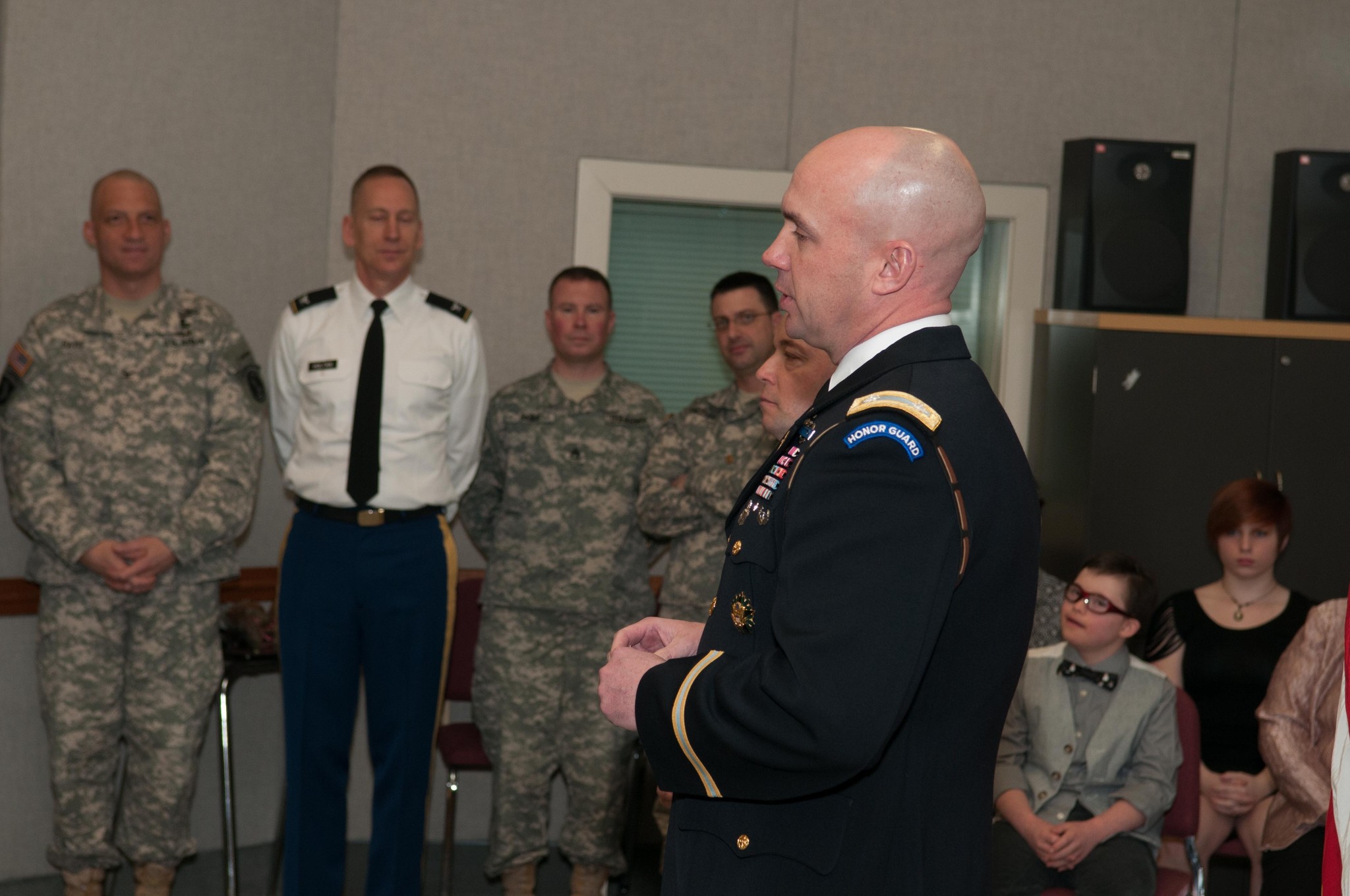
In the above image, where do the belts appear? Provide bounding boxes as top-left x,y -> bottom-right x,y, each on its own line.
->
297,497 -> 442,528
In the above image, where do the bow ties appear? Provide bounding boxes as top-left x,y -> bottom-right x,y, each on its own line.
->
1056,660 -> 1118,691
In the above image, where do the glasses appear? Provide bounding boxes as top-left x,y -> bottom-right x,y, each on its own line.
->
708,310 -> 773,331
1064,583 -> 1138,619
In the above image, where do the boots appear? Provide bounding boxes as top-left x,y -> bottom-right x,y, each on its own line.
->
502,866 -> 536,896
134,863 -> 176,896
63,869 -> 104,896
570,863 -> 609,896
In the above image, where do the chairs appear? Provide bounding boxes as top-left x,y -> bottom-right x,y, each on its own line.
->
435,569 -> 493,895
1041,688 -> 1203,896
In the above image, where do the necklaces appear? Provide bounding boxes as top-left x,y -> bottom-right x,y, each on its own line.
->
1219,580 -> 1275,620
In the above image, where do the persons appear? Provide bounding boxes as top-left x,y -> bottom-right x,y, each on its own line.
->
0,170 -> 269,894
272,165 -> 489,896
988,553 -> 1184,896
1140,474 -> 1325,896
1253,594 -> 1349,895
754,311 -> 837,440
461,268 -> 674,896
633,270 -> 783,825
594,127 -> 1048,896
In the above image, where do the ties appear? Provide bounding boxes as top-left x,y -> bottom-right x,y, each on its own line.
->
813,377 -> 831,404
347,300 -> 389,504
